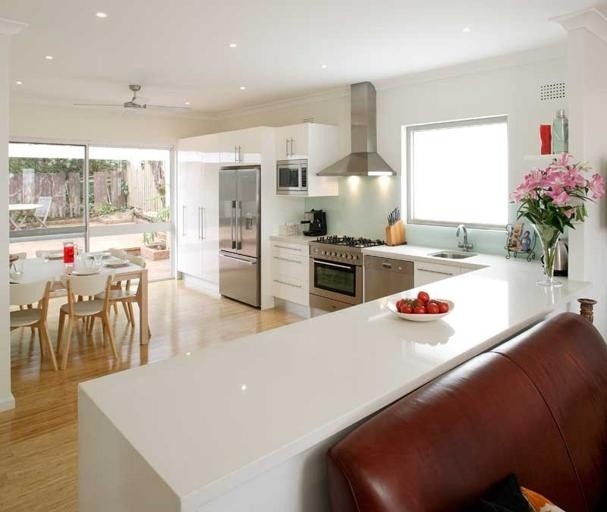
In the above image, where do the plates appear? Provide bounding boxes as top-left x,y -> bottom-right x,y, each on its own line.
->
9,250 -> 128,283
387,298 -> 454,322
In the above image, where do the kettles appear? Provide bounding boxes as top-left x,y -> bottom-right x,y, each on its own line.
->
548,238 -> 568,276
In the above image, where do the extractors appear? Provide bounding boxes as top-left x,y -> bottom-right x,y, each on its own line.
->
312,82 -> 395,176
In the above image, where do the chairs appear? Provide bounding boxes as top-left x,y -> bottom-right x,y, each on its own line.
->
9,248 -> 151,372
33,196 -> 53,228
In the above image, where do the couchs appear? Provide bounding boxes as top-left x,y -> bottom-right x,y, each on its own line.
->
328,314 -> 605,512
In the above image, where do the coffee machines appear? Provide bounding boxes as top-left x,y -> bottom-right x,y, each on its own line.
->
300,208 -> 326,237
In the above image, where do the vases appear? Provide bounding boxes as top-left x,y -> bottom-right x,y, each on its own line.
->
527,223 -> 563,289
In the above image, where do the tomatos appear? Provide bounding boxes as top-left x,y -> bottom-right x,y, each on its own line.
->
396,292 -> 448,314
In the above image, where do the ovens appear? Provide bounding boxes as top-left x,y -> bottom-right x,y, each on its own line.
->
307,256 -> 362,317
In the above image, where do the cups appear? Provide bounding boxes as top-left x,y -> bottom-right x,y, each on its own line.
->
63,240 -> 74,268
538,124 -> 551,156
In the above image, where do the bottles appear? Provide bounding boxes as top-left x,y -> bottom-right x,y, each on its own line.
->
551,109 -> 568,155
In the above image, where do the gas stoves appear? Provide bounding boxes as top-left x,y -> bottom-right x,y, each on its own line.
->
307,235 -> 384,260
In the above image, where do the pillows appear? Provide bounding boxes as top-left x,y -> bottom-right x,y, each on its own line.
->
513,482 -> 566,512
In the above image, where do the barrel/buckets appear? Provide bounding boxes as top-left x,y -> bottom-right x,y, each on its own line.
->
456,224 -> 468,251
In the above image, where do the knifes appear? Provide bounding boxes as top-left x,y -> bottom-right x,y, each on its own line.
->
386,207 -> 398,225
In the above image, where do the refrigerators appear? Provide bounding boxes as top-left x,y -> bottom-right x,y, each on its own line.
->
218,167 -> 258,306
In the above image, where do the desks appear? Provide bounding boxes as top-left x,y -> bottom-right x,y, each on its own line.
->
8,204 -> 43,229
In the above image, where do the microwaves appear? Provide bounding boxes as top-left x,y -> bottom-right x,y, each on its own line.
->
275,160 -> 307,194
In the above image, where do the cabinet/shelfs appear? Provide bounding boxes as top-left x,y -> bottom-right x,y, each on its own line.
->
175,134 -> 221,286
276,123 -> 338,160
414,259 -> 473,303
270,239 -> 307,306
222,127 -> 276,165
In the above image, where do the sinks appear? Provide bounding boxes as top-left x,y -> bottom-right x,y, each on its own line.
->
429,249 -> 477,261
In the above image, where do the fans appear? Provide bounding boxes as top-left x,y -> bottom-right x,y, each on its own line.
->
73,86 -> 190,112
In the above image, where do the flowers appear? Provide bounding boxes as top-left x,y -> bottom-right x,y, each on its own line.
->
508,153 -> 604,276
